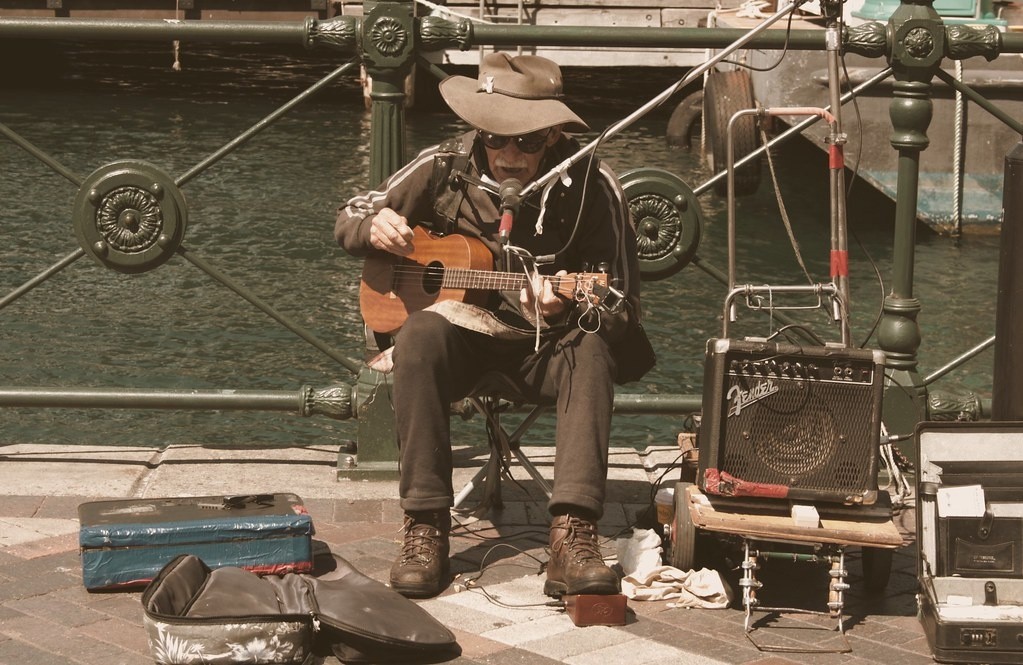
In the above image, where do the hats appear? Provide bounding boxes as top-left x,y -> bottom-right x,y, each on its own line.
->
439,51 -> 591,136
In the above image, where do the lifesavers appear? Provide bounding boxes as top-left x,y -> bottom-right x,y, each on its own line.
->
665,70 -> 761,200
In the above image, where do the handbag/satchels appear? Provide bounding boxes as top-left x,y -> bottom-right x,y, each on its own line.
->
140,552 -> 456,664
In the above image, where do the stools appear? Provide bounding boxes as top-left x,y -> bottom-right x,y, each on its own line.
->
454,370 -> 552,510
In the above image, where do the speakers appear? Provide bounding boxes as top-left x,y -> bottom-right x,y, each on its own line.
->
695,337 -> 885,504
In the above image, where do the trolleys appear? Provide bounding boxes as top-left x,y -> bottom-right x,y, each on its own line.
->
658,106 -> 919,596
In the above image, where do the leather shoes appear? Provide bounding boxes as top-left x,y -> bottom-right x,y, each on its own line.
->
388,509 -> 450,597
543,512 -> 621,599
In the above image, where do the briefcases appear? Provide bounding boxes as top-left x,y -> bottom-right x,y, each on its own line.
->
76,492 -> 316,593
914,421 -> 1023,665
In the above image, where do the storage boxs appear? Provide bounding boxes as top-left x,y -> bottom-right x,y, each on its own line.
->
77,492 -> 316,590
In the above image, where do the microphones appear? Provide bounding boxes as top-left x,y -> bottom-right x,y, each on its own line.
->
498,177 -> 523,244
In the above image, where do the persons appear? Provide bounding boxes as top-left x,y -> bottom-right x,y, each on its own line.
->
331,50 -> 657,596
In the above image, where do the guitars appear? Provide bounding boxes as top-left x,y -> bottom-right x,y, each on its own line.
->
357,222 -> 630,355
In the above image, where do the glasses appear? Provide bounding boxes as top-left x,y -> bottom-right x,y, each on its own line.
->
478,127 -> 552,153
